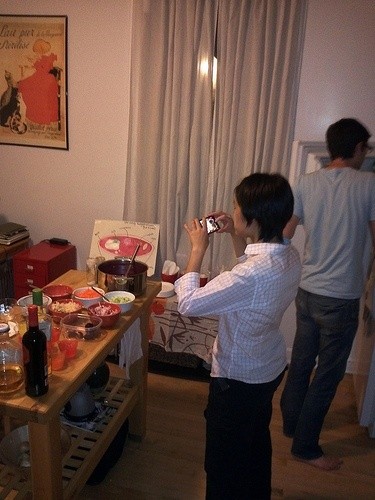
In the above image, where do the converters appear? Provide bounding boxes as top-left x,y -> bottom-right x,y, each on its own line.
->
49,237 -> 68,246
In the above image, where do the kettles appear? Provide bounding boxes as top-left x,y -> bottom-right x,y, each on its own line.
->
64,385 -> 96,421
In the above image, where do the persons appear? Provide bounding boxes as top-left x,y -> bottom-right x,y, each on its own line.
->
176,173 -> 303,500
280,118 -> 375,471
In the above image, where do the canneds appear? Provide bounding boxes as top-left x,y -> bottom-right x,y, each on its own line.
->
85,258 -> 99,285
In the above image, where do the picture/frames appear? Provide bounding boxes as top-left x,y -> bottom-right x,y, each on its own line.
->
289,140 -> 375,187
0,13 -> 69,151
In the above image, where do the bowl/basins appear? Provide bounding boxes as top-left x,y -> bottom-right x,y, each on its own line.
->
57,331 -> 79,358
88,303 -> 120,327
42,285 -> 73,300
0,425 -> 71,470
72,287 -> 105,306
17,294 -> 52,310
0,298 -> 18,320
47,342 -> 67,370
60,313 -> 103,340
103,291 -> 135,313
48,298 -> 83,322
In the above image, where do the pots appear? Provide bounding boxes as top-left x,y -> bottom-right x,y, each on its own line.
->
97,257 -> 148,297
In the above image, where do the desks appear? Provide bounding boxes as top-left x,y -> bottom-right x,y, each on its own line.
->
153,294 -> 222,367
0,270 -> 163,500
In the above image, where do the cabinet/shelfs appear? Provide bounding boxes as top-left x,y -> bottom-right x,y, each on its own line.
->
12,242 -> 77,300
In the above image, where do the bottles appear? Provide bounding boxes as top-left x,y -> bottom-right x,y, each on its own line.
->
0,322 -> 24,395
32,289 -> 51,341
22,305 -> 49,396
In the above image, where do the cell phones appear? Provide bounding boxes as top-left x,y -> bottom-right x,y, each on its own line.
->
199,216 -> 221,235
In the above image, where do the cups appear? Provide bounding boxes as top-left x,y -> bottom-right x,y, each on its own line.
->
200,277 -> 208,287
86,258 -> 96,285
96,257 -> 105,268
161,273 -> 177,283
